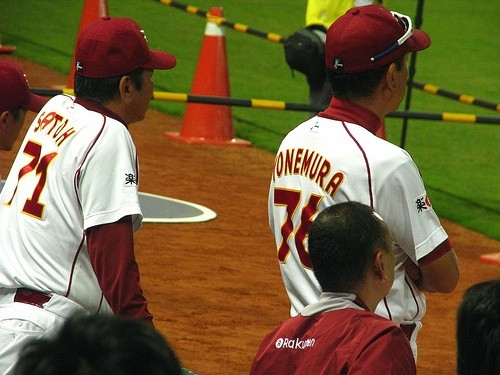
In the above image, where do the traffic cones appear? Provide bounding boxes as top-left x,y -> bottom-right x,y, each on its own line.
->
163,5 -> 254,147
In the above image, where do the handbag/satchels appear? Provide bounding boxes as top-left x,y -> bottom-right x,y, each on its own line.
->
283,29 -> 326,81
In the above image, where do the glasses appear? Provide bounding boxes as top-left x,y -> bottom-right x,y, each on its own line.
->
371,10 -> 413,62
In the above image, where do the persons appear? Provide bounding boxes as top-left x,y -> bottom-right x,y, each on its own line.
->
1,14 -> 180,375
265,2 -> 461,374
10,303 -> 186,375
1,55 -> 51,194
302,0 -> 378,119
454,276 -> 500,375
249,198 -> 418,375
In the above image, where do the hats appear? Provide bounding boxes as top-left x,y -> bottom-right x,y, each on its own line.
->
73,15 -> 176,81
0,60 -> 49,113
325,5 -> 430,76
